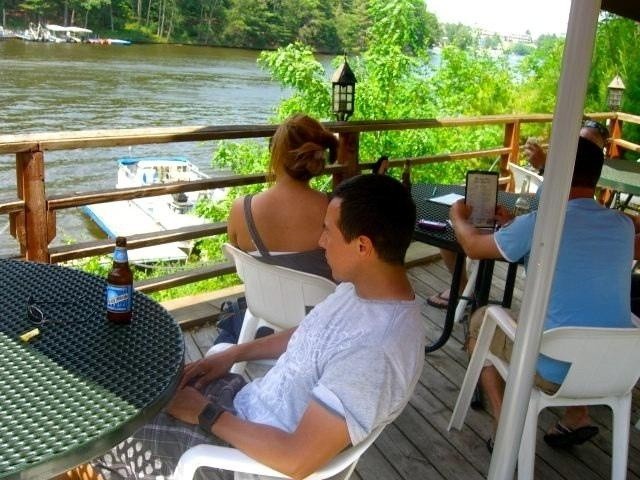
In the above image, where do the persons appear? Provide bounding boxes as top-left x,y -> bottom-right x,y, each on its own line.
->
448,133 -> 637,460
48,173 -> 429,480
620,209 -> 639,320
426,119 -> 612,309
211,110 -> 344,347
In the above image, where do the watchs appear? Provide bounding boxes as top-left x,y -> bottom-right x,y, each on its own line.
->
197,401 -> 224,438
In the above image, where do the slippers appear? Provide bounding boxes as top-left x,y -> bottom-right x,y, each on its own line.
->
486,434 -> 495,454
543,418 -> 599,449
426,292 -> 475,309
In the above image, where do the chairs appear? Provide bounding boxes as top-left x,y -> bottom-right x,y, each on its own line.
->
446,305 -> 639,479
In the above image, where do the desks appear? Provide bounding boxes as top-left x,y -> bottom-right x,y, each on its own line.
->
411,183 -> 542,353
596,158 -> 640,210
0,256 -> 187,480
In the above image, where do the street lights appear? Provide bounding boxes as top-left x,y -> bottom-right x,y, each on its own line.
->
608,74 -> 626,111
328,58 -> 357,123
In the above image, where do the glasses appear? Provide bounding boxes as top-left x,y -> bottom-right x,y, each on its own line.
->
268,137 -> 272,153
583,119 -> 609,145
26,294 -> 98,325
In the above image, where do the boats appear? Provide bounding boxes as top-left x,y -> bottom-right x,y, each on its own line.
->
78,155 -> 228,276
1,22 -> 131,45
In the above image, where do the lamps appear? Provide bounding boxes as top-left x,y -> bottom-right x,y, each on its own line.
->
607,70 -> 626,112
330,52 -> 357,121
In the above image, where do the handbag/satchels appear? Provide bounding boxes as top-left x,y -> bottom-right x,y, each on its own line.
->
213,296 -> 274,345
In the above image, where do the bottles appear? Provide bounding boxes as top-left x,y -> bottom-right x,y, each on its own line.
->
107,237 -> 133,323
511,175 -> 531,218
398,159 -> 412,193
